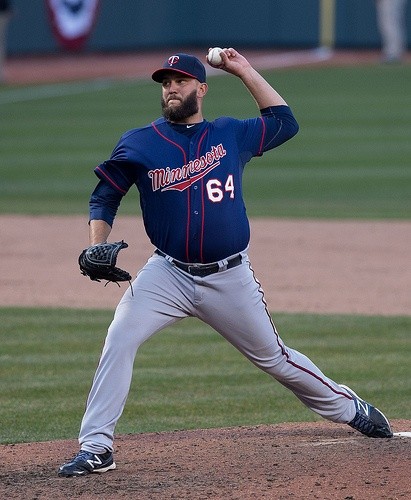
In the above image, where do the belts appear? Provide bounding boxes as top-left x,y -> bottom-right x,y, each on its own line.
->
154,249 -> 242,276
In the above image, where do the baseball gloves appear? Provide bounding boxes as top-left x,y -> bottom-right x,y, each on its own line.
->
77,238 -> 136,297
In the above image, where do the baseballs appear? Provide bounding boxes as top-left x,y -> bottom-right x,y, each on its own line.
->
208,47 -> 224,66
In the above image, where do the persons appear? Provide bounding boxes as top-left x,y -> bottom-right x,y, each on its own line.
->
60,49 -> 392,477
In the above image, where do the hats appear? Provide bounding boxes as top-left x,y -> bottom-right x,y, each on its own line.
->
151,54 -> 206,83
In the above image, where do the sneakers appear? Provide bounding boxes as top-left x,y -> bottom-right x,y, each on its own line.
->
61,449 -> 115,475
338,384 -> 393,438
